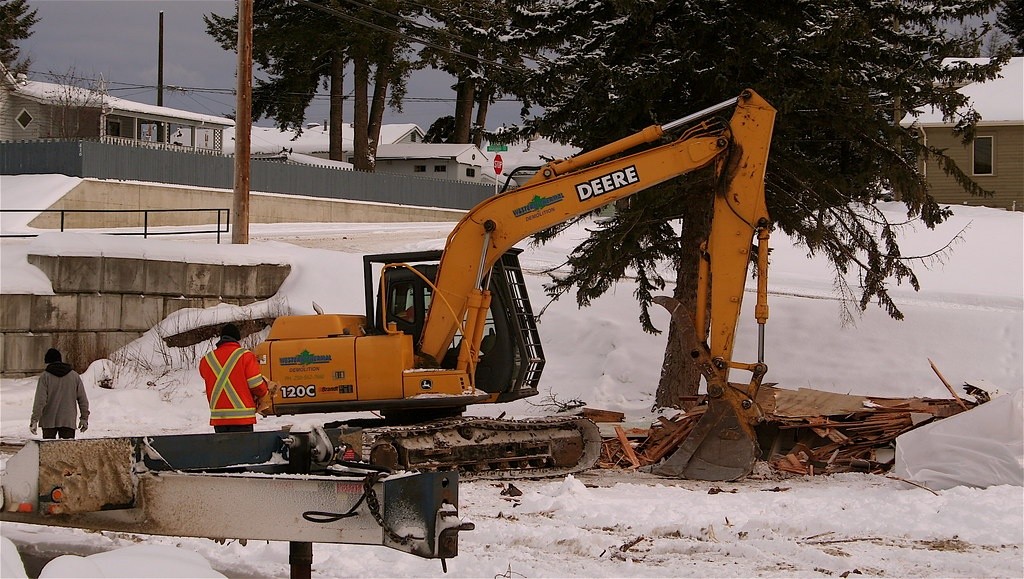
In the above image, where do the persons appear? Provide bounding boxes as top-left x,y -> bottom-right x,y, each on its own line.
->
30,348 -> 90,440
199,324 -> 268,433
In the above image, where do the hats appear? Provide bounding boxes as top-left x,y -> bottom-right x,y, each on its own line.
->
44,348 -> 62,363
220,324 -> 241,341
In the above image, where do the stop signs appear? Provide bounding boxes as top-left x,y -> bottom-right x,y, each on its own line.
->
494,154 -> 503,174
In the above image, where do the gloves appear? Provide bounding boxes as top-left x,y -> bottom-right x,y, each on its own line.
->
29,418 -> 38,434
78,419 -> 88,433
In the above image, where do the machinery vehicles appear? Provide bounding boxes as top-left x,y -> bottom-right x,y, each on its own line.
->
252,87 -> 778,485
